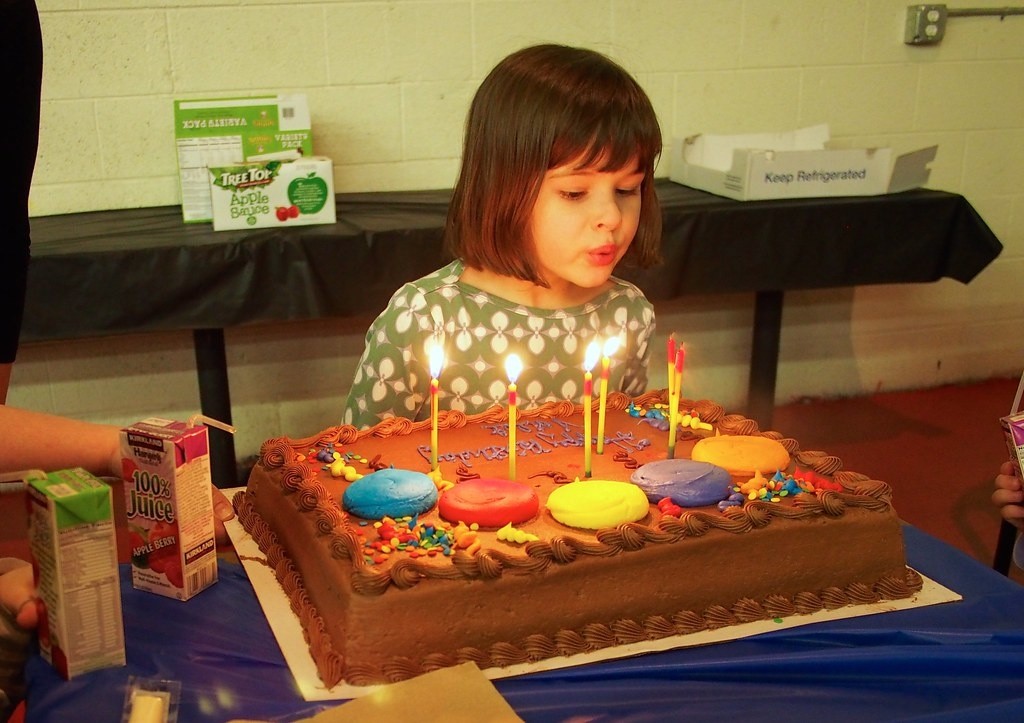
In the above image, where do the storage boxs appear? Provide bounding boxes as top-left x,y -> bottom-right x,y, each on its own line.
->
173,93 -> 311,223
207,156 -> 336,231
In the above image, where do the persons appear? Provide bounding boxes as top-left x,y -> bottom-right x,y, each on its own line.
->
1,406 -> 236,650
990,460 -> 1023,538
341,45 -> 663,437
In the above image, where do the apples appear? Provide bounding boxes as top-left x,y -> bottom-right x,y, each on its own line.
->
122,457 -> 184,589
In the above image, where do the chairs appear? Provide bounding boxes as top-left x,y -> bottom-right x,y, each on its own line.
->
990,371 -> 1024,577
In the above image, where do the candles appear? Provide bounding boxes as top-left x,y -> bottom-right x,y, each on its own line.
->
425,344 -> 442,488
582,344 -> 600,478
597,336 -> 619,454
667,332 -> 677,442
666,341 -> 684,459
503,353 -> 523,481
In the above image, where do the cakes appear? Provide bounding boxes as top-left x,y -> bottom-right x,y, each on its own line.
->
230,387 -> 923,690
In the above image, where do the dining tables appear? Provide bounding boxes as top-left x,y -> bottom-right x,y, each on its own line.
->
25,487 -> 1024,723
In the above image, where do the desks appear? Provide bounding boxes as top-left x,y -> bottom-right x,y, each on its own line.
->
21,177 -> 1001,486
